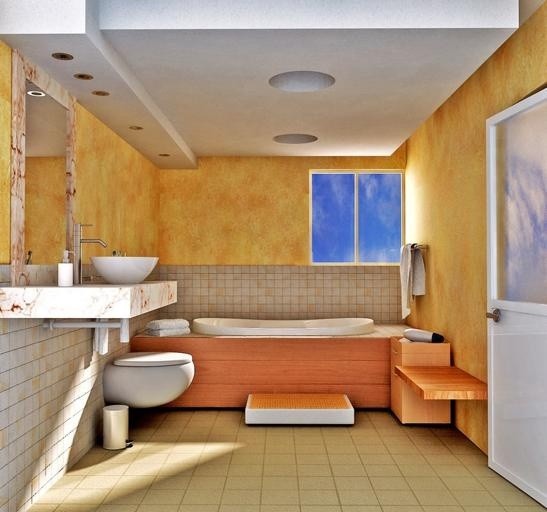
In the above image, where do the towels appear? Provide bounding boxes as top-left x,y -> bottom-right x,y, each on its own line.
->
399,244 -> 426,320
145,319 -> 190,336
93,319 -> 108,355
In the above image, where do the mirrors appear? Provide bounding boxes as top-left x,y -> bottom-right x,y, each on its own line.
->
10,47 -> 76,286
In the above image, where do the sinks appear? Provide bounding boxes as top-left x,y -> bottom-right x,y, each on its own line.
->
91,256 -> 160,283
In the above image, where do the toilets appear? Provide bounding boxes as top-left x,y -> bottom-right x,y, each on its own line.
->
103,351 -> 195,407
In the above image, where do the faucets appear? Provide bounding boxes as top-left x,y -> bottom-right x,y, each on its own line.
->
69,224 -> 107,285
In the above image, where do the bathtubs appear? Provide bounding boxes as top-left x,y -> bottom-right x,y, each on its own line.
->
192,317 -> 374,335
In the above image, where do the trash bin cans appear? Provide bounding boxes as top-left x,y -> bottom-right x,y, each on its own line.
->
103,405 -> 134,451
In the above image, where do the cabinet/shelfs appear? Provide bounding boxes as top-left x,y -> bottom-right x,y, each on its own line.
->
391,335 -> 452,424
